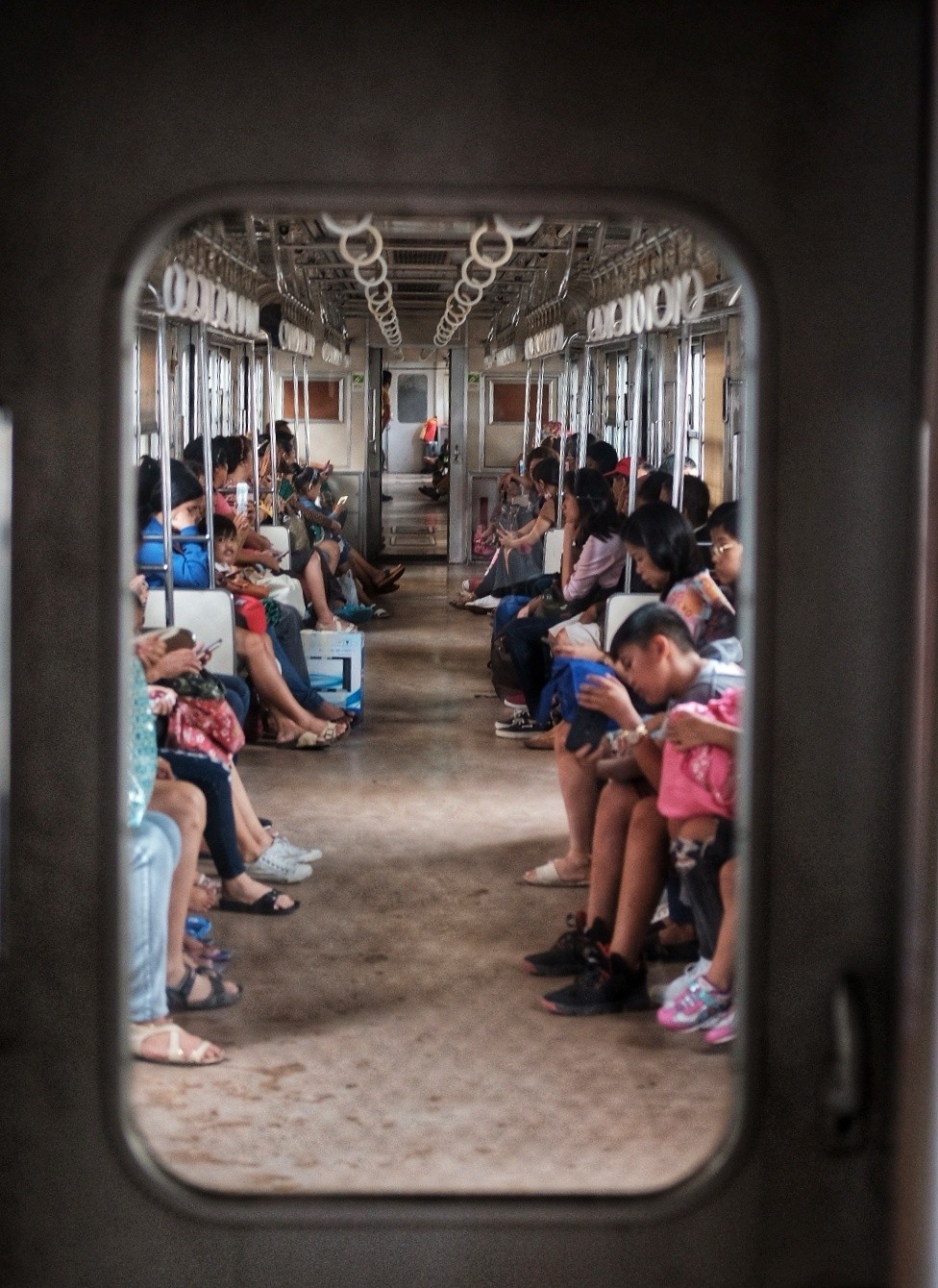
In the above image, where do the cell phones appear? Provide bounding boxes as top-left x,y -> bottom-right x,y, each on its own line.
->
338,496 -> 349,504
276,549 -> 291,562
198,640 -> 222,660
224,569 -> 242,577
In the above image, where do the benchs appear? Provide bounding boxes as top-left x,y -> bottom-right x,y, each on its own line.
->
139,525 -> 293,677
543,527 -> 659,655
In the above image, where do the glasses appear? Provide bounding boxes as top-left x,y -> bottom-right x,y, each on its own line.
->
708,541 -> 738,559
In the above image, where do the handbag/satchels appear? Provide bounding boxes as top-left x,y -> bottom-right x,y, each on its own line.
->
279,501 -> 310,553
657,686 -> 744,822
126,655 -> 158,823
536,655 -> 645,749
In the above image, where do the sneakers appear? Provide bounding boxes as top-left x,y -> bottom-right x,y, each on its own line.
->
538,941 -> 650,1016
450,576 -> 557,751
522,910 -> 615,976
646,957 -> 713,1007
705,1007 -> 741,1046
657,975 -> 731,1033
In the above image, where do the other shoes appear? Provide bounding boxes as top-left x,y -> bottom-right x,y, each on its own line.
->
647,919 -> 666,934
644,934 -> 700,961
419,486 -> 439,500
382,494 -> 393,501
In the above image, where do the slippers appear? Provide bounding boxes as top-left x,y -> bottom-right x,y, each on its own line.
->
274,563 -> 406,751
525,860 -> 591,886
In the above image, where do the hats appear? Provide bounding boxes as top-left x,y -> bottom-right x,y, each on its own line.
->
603,457 -> 645,478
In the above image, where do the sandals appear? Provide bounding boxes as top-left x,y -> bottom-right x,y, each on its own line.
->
126,817 -> 324,1067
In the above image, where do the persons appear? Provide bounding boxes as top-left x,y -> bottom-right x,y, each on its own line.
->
418,420 -> 743,1046
123,368 -> 405,1068
